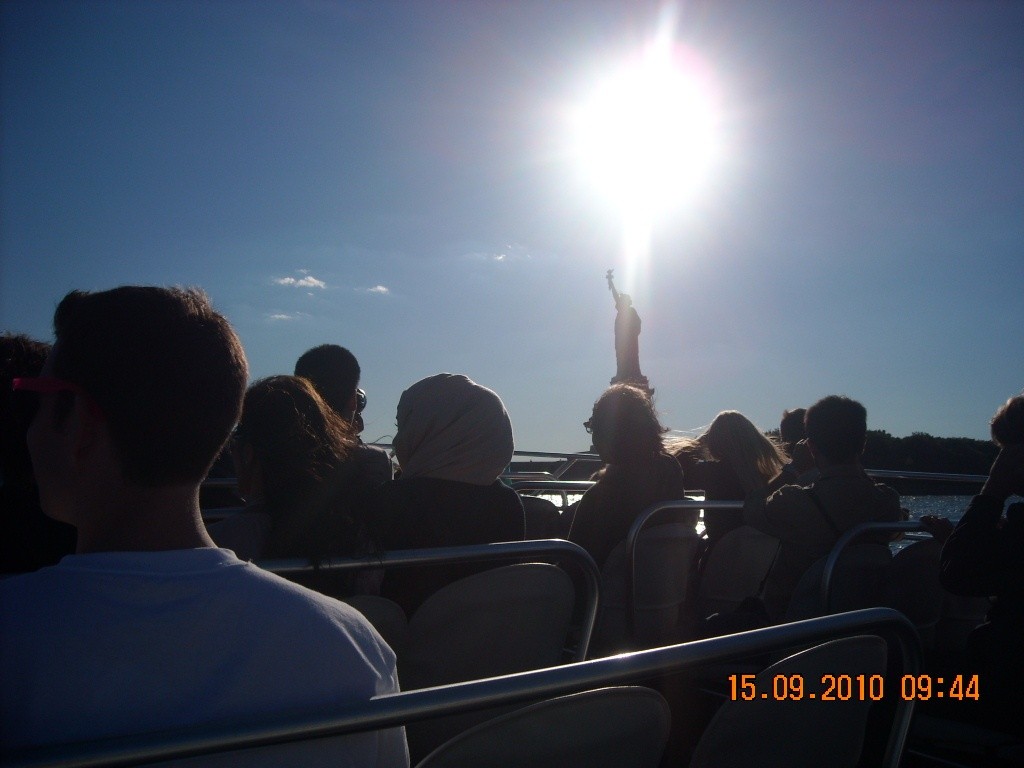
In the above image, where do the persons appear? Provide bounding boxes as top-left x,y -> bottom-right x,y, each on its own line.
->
606,271 -> 642,379
354,374 -> 526,619
743,396 -> 893,637
205,375 -> 382,601
680,413 -> 781,505
562,384 -> 685,642
0,283 -> 413,767
0,333 -> 78,572
772,406 -> 815,491
919,397 -> 1024,724
294,345 -> 400,526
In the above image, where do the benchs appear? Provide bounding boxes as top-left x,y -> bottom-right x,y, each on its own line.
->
0,471 -> 964,768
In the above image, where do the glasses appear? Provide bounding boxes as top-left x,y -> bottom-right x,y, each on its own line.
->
584,422 -> 594,431
12,376 -> 105,428
